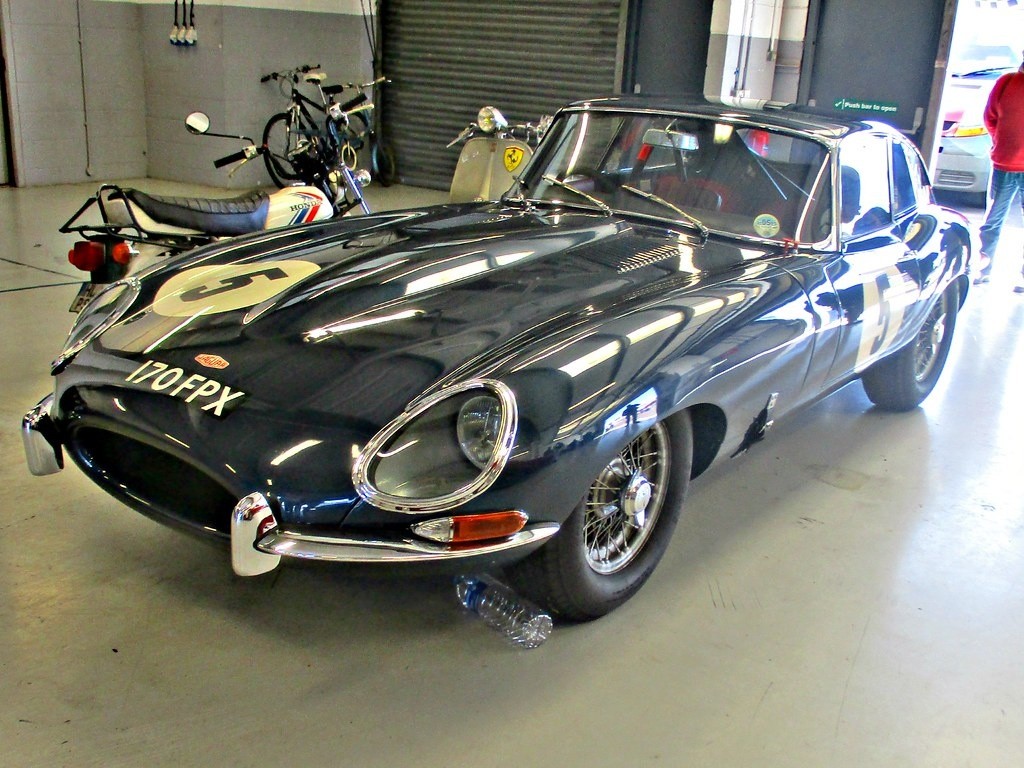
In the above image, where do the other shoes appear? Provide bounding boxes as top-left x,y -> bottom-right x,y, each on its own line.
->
973,275 -> 991,283
1013,277 -> 1024,293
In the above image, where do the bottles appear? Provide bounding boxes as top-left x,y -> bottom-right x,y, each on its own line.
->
454,574 -> 553,650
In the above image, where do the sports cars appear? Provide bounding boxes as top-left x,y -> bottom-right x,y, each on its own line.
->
21,92 -> 974,624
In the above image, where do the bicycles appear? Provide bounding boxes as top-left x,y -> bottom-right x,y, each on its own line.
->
260,62 -> 398,190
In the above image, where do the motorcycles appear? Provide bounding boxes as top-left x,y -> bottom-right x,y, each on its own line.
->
57,92 -> 376,315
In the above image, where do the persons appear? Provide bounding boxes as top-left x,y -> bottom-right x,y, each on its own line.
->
971,47 -> 1024,294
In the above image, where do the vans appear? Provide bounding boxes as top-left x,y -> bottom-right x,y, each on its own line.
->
932,7 -> 1024,206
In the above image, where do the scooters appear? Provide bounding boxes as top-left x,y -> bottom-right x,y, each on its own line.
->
445,105 -> 555,208
